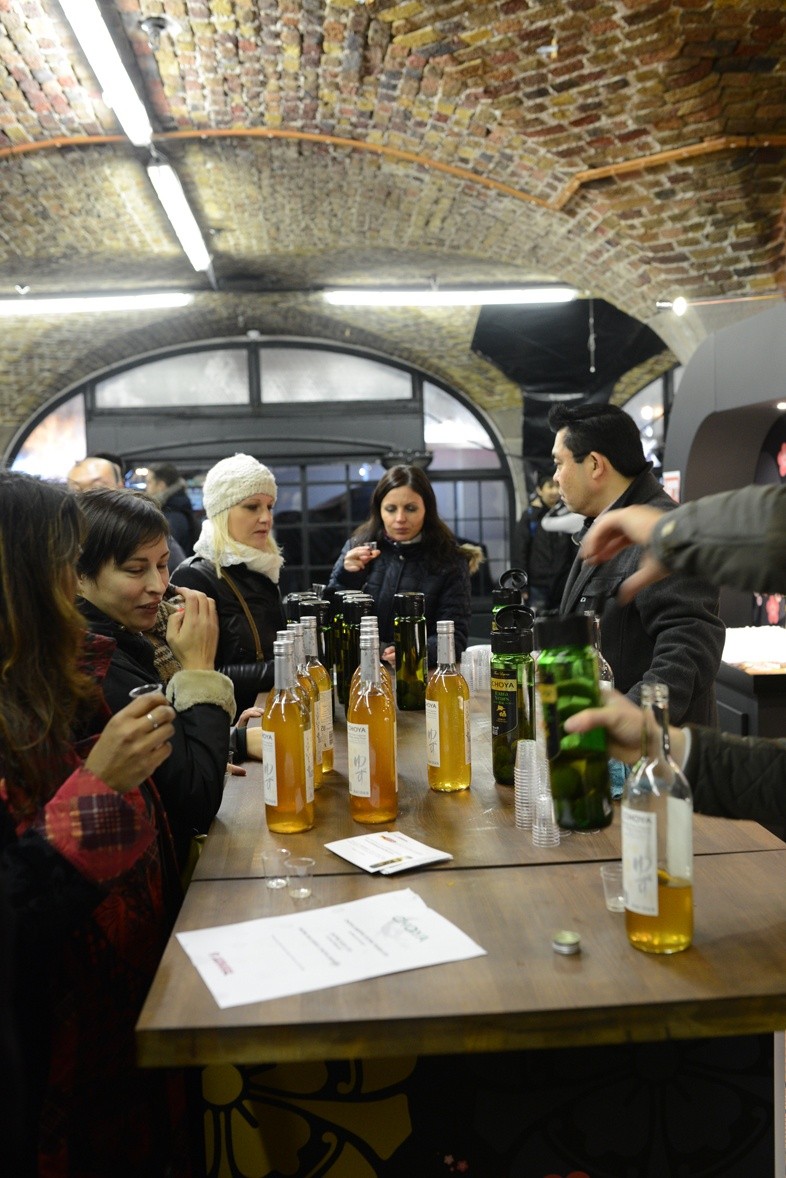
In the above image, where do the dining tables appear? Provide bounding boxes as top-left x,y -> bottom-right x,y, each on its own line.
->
138,847 -> 786,1178
191,683 -> 785,883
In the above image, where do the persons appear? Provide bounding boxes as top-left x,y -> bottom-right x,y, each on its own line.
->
164,452 -> 290,726
144,465 -> 200,556
67,489 -> 237,885
129,489 -> 264,777
510,472 -> 561,616
562,483 -> 786,842
0,471 -> 175,1175
66,456 -> 187,577
327,464 -> 485,668
548,404 -> 726,725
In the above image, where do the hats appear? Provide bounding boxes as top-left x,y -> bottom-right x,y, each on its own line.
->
202,452 -> 277,518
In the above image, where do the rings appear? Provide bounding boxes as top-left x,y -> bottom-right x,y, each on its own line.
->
146,712 -> 160,731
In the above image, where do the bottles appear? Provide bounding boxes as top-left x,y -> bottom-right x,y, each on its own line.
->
263,568 -> 696,954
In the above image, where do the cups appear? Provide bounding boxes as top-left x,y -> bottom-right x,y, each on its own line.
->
364,541 -> 376,558
130,683 -> 161,703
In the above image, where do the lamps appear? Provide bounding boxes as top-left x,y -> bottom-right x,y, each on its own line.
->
146,157 -> 212,273
57,0 -> 157,148
